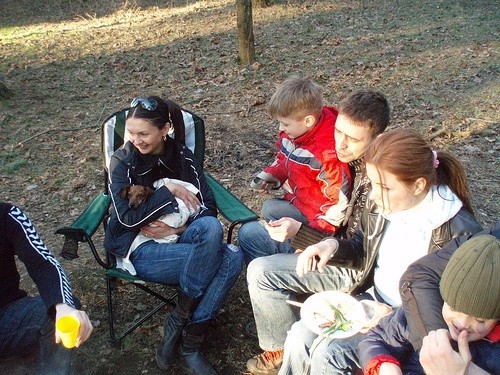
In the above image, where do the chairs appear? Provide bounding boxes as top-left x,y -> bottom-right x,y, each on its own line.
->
53,106 -> 260,345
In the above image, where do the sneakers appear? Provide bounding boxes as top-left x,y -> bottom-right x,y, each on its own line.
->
246,349 -> 284,374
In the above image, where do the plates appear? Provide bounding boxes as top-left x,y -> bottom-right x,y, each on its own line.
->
300,291 -> 366,338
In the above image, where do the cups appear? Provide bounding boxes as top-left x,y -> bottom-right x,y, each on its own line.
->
56,316 -> 81,348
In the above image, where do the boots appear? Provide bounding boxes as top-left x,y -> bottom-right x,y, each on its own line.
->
176,313 -> 219,375
155,287 -> 204,372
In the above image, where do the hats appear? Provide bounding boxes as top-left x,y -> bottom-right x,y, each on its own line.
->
439,234 -> 500,319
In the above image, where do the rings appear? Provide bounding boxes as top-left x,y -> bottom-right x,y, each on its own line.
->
146,233 -> 147,237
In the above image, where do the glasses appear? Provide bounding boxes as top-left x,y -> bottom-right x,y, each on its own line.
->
130,96 -> 160,111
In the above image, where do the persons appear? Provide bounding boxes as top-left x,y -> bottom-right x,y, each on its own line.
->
358,233 -> 500,375
103,96 -> 243,375
398,221 -> 500,375
0,202 -> 93,375
277,126 -> 485,375
237,91 -> 392,375
251,76 -> 355,235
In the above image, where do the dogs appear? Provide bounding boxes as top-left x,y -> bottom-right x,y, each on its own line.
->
120,183 -> 201,228
